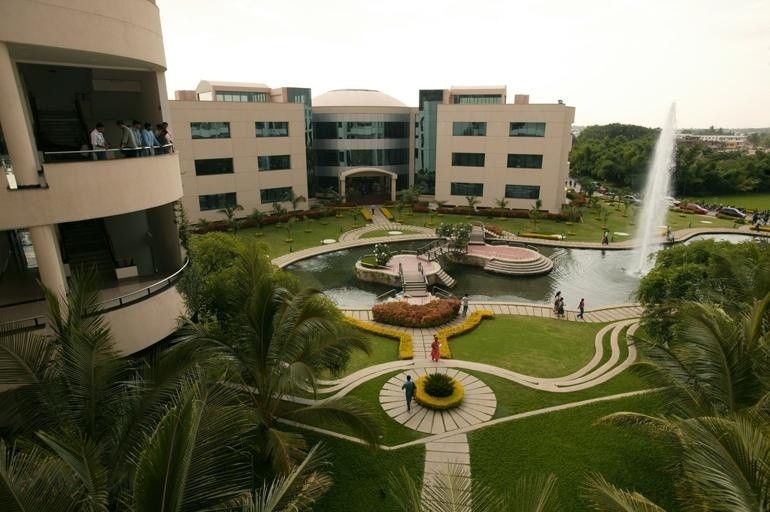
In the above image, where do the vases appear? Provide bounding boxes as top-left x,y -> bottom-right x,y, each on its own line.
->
381,260 -> 386,266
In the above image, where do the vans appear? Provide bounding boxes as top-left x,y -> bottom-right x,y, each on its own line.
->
590,182 -> 600,190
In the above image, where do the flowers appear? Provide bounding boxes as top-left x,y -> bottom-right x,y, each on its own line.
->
373,242 -> 392,262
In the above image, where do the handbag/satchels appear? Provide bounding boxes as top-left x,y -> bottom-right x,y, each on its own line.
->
412,382 -> 416,397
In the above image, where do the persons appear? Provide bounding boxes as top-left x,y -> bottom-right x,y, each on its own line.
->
91,123 -> 111,161
553,290 -> 566,319
665,223 -> 675,243
115,120 -> 176,155
577,298 -> 585,318
602,231 -> 609,245
431,336 -> 440,361
401,375 -> 415,412
461,293 -> 473,317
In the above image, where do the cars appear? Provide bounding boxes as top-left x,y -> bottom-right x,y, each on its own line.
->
680,204 -> 708,215
605,192 -> 616,196
598,187 -> 608,194
717,208 -> 747,219
623,195 -> 642,203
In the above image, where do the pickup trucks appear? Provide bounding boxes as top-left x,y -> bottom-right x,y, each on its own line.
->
566,188 -> 576,194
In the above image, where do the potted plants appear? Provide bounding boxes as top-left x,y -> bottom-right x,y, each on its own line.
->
413,371 -> 465,410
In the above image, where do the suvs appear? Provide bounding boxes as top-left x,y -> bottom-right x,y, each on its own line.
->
664,197 -> 680,206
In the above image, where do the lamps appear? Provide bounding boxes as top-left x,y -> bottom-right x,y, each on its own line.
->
1,154 -> 19,190
